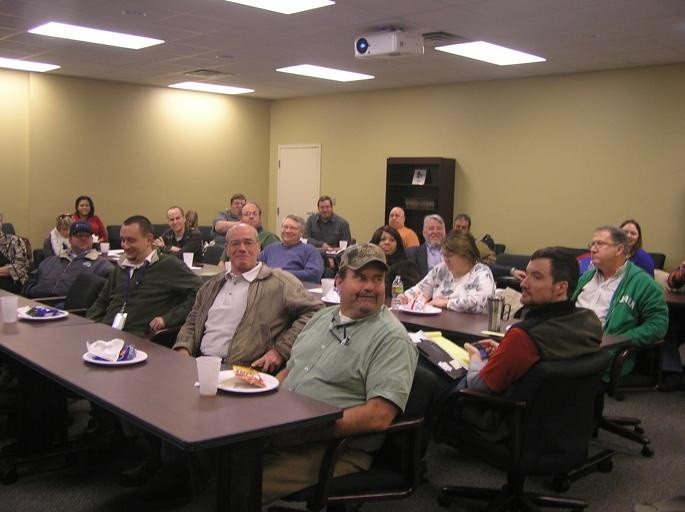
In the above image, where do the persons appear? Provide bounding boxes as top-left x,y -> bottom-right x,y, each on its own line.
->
175,241 -> 419,512
370,208 -> 526,313
620,220 -> 655,280
570,226 -> 670,392
403,247 -> 602,458
1,196 -> 204,350
666,260 -> 685,375
210,194 -> 352,284
116,224 -> 326,512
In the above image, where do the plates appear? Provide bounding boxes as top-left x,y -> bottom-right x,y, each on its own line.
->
82,350 -> 149,367
400,303 -> 443,316
217,368 -> 280,396
320,295 -> 339,305
17,309 -> 68,322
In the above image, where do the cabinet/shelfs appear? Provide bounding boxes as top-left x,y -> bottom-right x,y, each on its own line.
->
385,157 -> 455,243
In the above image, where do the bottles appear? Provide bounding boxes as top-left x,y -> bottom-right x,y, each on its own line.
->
391,275 -> 404,315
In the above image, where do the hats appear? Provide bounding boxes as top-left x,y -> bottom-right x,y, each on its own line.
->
339,243 -> 391,274
69,221 -> 94,234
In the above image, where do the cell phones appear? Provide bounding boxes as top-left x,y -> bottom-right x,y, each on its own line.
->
473,342 -> 489,360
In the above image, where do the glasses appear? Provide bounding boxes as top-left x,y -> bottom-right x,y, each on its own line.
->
588,240 -> 618,249
59,214 -> 73,220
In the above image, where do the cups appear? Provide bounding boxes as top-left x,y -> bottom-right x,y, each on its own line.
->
486,295 -> 512,332
320,278 -> 334,296
338,240 -> 347,251
182,252 -> 193,269
0,295 -> 19,324
195,355 -> 223,397
99,242 -> 109,258
300,238 -> 307,245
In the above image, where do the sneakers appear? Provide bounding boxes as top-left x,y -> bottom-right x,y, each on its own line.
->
66,420 -> 124,450
115,455 -> 156,488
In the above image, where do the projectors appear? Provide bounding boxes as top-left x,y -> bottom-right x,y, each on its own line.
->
353,30 -> 423,59
